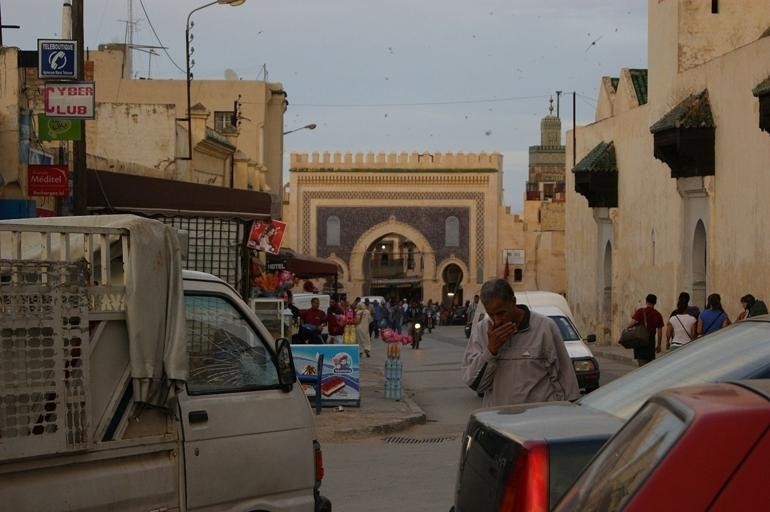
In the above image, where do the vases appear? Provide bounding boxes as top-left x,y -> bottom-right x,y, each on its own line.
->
267,291 -> 276,298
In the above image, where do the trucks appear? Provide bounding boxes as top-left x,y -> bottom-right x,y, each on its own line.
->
249,289 -> 330,345
0,214 -> 334,512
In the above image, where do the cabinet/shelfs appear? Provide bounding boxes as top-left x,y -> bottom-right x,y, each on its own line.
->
248,298 -> 285,347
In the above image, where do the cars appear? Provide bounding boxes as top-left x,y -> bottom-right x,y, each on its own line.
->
465,321 -> 474,337
455,307 -> 465,318
550,378 -> 769,511
446,309 -> 769,511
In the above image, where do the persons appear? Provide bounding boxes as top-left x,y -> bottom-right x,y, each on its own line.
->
325,295 -> 454,358
467,295 -> 479,323
462,278 -> 581,408
666,299 -> 697,351
669,292 -> 700,319
696,293 -> 729,338
737,294 -> 755,320
618,294 -> 665,367
288,294 -> 327,344
260,225 -> 276,250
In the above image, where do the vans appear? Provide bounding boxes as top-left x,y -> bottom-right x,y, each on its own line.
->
358,294 -> 385,306
473,290 -> 600,394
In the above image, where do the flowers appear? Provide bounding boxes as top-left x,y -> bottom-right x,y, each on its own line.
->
254,269 -> 294,292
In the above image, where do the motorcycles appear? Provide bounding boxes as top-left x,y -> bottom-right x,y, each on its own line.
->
409,315 -> 424,350
425,311 -> 435,333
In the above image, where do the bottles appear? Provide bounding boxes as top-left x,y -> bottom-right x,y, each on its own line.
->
344,324 -> 356,344
331,311 -> 342,322
345,309 -> 353,324
384,341 -> 404,400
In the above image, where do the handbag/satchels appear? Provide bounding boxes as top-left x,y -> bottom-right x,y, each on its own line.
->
619,325 -> 652,348
